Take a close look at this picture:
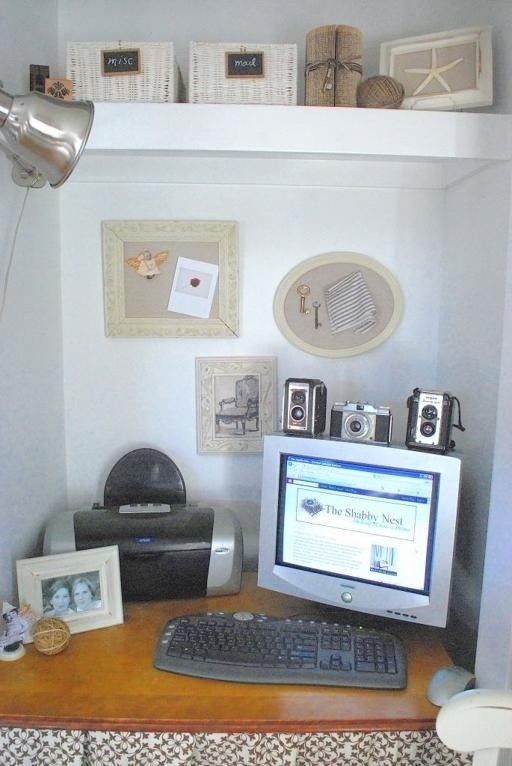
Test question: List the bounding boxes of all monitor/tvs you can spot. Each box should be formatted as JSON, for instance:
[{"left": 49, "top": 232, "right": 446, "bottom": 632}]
[{"left": 257, "top": 432, "right": 466, "bottom": 629}]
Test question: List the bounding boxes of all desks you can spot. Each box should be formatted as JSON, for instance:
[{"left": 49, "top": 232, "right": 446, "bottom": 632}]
[{"left": 1, "top": 570, "right": 466, "bottom": 743}]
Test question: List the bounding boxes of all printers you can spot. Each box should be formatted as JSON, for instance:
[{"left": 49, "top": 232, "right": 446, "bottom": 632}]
[{"left": 43, "top": 448, "right": 243, "bottom": 601}]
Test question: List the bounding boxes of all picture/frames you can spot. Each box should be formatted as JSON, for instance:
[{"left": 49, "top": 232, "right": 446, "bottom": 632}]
[
  {"left": 100, "top": 219, "right": 242, "bottom": 341},
  {"left": 13, "top": 545, "right": 130, "bottom": 646},
  {"left": 193, "top": 355, "right": 280, "bottom": 456},
  {"left": 377, "top": 22, "right": 494, "bottom": 111}
]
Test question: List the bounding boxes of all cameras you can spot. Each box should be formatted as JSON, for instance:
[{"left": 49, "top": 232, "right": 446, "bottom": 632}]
[{"left": 330, "top": 401, "right": 393, "bottom": 445}]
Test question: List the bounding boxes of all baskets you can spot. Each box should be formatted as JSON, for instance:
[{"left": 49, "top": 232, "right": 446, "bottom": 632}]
[
  {"left": 66, "top": 39, "right": 179, "bottom": 108},
  {"left": 187, "top": 40, "right": 298, "bottom": 107}
]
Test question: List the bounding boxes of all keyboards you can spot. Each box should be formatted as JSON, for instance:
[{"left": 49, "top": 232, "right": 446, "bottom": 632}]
[{"left": 152, "top": 612, "right": 407, "bottom": 689}]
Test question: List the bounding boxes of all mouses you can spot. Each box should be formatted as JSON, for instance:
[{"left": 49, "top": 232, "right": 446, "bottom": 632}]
[{"left": 426, "top": 664, "right": 475, "bottom": 708}]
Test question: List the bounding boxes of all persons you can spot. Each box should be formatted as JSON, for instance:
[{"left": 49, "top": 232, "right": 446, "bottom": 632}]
[
  {"left": 0, "top": 600, "right": 33, "bottom": 663},
  {"left": 44, "top": 580, "right": 75, "bottom": 617},
  {"left": 71, "top": 578, "right": 101, "bottom": 613}
]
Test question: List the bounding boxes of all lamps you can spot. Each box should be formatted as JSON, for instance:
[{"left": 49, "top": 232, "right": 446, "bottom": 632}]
[{"left": 1, "top": 84, "right": 101, "bottom": 192}]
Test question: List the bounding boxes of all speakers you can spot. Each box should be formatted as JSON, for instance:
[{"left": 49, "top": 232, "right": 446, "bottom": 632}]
[
  {"left": 283, "top": 378, "right": 326, "bottom": 436},
  {"left": 407, "top": 388, "right": 454, "bottom": 455}
]
